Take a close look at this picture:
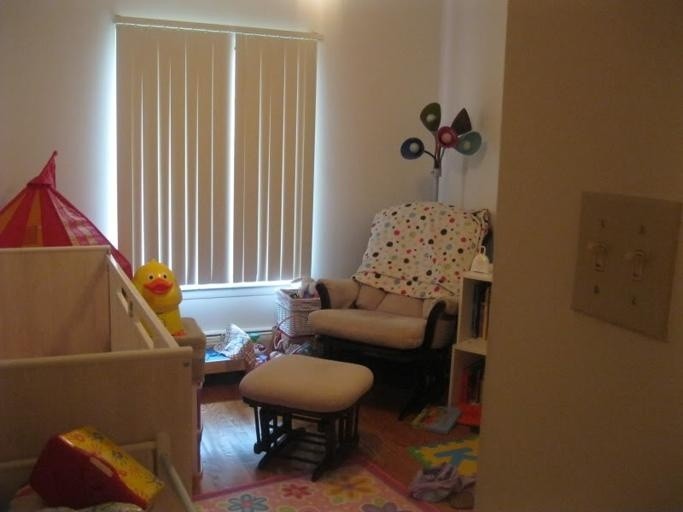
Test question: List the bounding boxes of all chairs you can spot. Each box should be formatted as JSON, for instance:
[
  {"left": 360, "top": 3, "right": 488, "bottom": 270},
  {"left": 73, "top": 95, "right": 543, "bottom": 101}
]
[{"left": 316, "top": 202, "right": 491, "bottom": 421}]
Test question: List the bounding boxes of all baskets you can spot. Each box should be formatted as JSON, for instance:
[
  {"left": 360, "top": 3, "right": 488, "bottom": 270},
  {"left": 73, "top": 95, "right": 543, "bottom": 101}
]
[{"left": 276, "top": 289, "right": 321, "bottom": 337}]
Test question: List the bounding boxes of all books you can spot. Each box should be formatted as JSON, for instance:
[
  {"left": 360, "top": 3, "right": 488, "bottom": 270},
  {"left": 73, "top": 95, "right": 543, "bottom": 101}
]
[
  {"left": 471, "top": 284, "right": 489, "bottom": 343},
  {"left": 465, "top": 355, "right": 486, "bottom": 406},
  {"left": 408, "top": 402, "right": 463, "bottom": 434}
]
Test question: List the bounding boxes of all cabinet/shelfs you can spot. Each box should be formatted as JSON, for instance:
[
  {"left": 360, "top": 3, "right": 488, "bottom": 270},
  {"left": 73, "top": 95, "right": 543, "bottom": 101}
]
[{"left": 446, "top": 270, "right": 491, "bottom": 427}]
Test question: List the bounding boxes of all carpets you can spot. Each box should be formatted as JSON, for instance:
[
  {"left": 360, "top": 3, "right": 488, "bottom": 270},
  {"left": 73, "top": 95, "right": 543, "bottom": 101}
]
[{"left": 191, "top": 453, "right": 442, "bottom": 511}]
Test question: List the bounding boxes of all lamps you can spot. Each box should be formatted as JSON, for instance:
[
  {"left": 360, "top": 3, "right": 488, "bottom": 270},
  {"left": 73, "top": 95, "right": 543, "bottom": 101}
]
[{"left": 399, "top": 101, "right": 482, "bottom": 203}]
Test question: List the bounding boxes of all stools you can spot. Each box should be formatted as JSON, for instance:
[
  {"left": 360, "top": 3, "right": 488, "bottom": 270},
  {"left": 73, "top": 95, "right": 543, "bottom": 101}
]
[{"left": 238, "top": 353, "right": 375, "bottom": 482}]
